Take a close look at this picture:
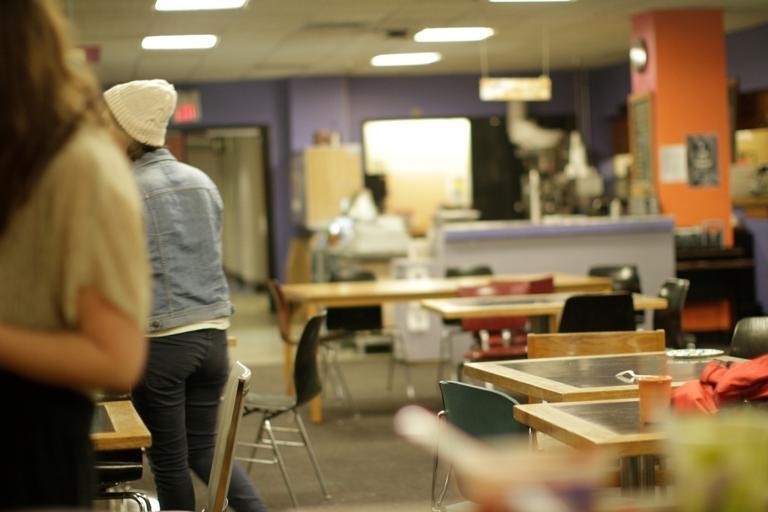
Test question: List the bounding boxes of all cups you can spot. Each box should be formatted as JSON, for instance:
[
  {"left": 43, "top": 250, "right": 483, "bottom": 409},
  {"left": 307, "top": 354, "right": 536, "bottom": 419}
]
[{"left": 637, "top": 375, "right": 673, "bottom": 425}]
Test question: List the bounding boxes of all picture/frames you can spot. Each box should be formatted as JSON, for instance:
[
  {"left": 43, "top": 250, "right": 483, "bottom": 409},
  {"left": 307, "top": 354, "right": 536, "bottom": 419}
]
[{"left": 627, "top": 91, "right": 656, "bottom": 198}]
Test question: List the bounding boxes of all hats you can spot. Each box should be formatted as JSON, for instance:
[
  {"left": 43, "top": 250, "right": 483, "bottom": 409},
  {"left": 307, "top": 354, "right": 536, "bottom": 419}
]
[{"left": 103, "top": 79, "right": 177, "bottom": 147}]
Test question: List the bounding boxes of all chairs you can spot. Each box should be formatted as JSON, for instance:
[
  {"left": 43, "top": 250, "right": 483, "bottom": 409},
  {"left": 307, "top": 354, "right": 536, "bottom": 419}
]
[
  {"left": 322, "top": 269, "right": 410, "bottom": 408},
  {"left": 589, "top": 265, "right": 646, "bottom": 327},
  {"left": 196, "top": 357, "right": 252, "bottom": 511},
  {"left": 223, "top": 311, "right": 330, "bottom": 511},
  {"left": 559, "top": 294, "right": 640, "bottom": 332},
  {"left": 436, "top": 265, "right": 504, "bottom": 396},
  {"left": 651, "top": 276, "right": 691, "bottom": 350},
  {"left": 430, "top": 372, "right": 536, "bottom": 512},
  {"left": 525, "top": 330, "right": 669, "bottom": 358},
  {"left": 270, "top": 274, "right": 362, "bottom": 422}
]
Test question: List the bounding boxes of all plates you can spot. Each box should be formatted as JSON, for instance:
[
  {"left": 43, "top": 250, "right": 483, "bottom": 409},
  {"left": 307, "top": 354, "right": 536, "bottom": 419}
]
[{"left": 666, "top": 348, "right": 725, "bottom": 359}]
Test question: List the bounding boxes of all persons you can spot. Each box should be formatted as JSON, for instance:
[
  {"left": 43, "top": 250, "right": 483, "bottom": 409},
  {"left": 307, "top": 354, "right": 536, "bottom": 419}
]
[
  {"left": 325, "top": 175, "right": 404, "bottom": 252},
  {"left": 0, "top": 0, "right": 152, "bottom": 512},
  {"left": 101, "top": 78, "right": 268, "bottom": 512}
]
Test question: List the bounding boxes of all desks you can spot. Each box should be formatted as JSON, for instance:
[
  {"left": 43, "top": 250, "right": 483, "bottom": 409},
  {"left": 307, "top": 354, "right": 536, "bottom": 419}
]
[
  {"left": 512, "top": 397, "right": 741, "bottom": 512},
  {"left": 461, "top": 347, "right": 746, "bottom": 401}
]
